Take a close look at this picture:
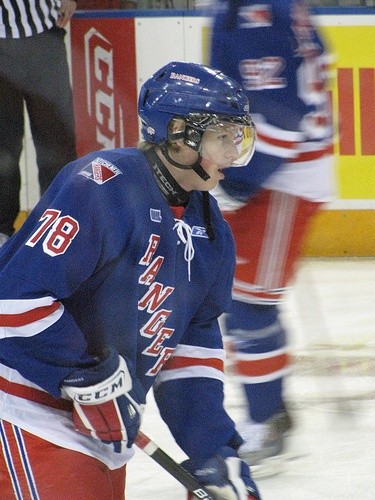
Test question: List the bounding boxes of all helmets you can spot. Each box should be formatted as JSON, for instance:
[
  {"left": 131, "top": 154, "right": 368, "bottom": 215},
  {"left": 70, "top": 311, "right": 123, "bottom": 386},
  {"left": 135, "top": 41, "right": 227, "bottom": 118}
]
[{"left": 138, "top": 60, "right": 257, "bottom": 169}]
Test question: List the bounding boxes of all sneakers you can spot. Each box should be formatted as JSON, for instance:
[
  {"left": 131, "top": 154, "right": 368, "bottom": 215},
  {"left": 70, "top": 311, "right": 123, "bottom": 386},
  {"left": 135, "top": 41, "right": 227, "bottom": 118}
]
[{"left": 234, "top": 411, "right": 312, "bottom": 480}]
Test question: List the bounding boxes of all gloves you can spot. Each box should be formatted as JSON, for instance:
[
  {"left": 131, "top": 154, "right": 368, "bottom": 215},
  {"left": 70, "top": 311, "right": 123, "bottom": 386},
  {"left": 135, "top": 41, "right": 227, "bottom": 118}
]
[
  {"left": 182, "top": 457, "right": 260, "bottom": 500},
  {"left": 63, "top": 344, "right": 141, "bottom": 454}
]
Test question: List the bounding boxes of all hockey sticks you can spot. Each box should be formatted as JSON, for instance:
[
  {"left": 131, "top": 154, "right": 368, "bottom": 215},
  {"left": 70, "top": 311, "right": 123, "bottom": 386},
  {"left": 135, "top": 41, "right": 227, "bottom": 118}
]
[{"left": 135, "top": 430, "right": 216, "bottom": 500}]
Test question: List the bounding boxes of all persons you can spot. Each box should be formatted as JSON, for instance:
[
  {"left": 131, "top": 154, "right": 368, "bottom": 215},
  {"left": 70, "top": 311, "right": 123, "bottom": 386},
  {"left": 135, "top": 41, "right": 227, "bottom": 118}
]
[
  {"left": 0, "top": 63, "right": 261, "bottom": 500},
  {"left": 193, "top": 0, "right": 334, "bottom": 485},
  {"left": 0, "top": 0, "right": 78, "bottom": 245}
]
